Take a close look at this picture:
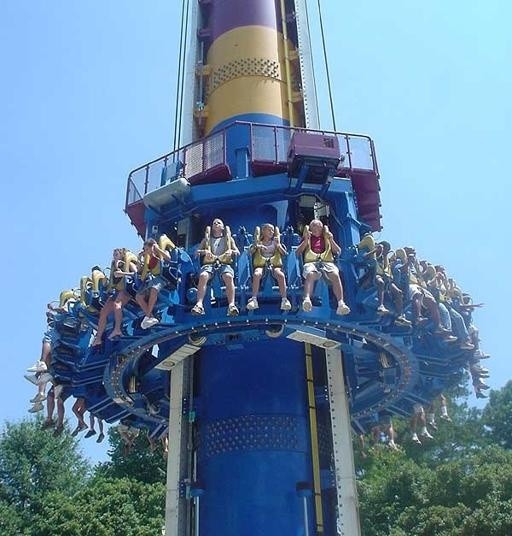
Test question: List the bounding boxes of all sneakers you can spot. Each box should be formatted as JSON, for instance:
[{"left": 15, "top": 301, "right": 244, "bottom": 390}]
[
  {"left": 24, "top": 361, "right": 169, "bottom": 458},
  {"left": 280, "top": 300, "right": 291, "bottom": 310},
  {"left": 246, "top": 298, "right": 259, "bottom": 310},
  {"left": 358, "top": 308, "right": 491, "bottom": 460},
  {"left": 191, "top": 305, "right": 205, "bottom": 315},
  {"left": 302, "top": 297, "right": 313, "bottom": 313},
  {"left": 227, "top": 306, "right": 239, "bottom": 316},
  {"left": 336, "top": 304, "right": 351, "bottom": 316},
  {"left": 141, "top": 316, "right": 159, "bottom": 329}
]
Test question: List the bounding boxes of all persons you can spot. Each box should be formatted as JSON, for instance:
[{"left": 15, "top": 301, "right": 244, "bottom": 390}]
[
  {"left": 354, "top": 241, "right": 491, "bottom": 459},
  {"left": 191, "top": 218, "right": 240, "bottom": 317},
  {"left": 24, "top": 248, "right": 168, "bottom": 460},
  {"left": 295, "top": 220, "right": 351, "bottom": 315},
  {"left": 247, "top": 223, "right": 292, "bottom": 311},
  {"left": 135, "top": 238, "right": 171, "bottom": 330}
]
